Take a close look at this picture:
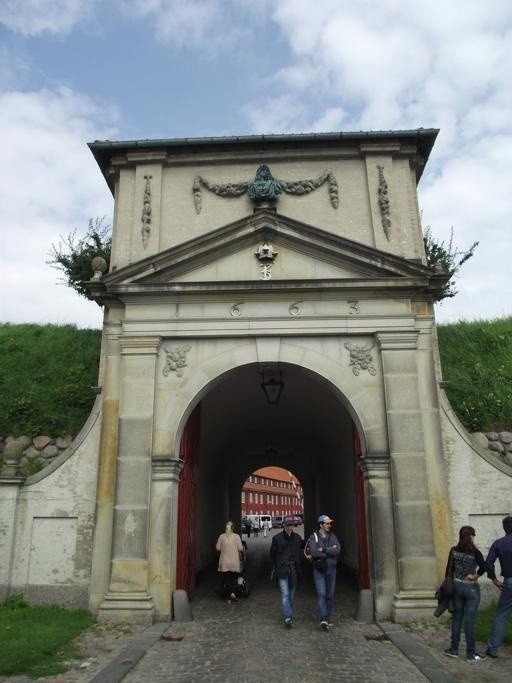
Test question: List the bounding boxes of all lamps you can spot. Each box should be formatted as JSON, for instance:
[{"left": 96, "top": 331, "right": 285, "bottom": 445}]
[
  {"left": 265, "top": 449, "right": 277, "bottom": 463},
  {"left": 261, "top": 369, "right": 286, "bottom": 404}
]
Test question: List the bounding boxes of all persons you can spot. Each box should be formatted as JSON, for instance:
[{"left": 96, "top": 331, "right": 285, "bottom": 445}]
[
  {"left": 486, "top": 516, "right": 512, "bottom": 658},
  {"left": 253, "top": 517, "right": 259, "bottom": 535},
  {"left": 254, "top": 519, "right": 259, "bottom": 538},
  {"left": 445, "top": 526, "right": 487, "bottom": 661},
  {"left": 263, "top": 519, "right": 269, "bottom": 536},
  {"left": 310, "top": 515, "right": 341, "bottom": 630},
  {"left": 246, "top": 518, "right": 252, "bottom": 538},
  {"left": 216, "top": 522, "right": 243, "bottom": 604},
  {"left": 270, "top": 517, "right": 302, "bottom": 625}
]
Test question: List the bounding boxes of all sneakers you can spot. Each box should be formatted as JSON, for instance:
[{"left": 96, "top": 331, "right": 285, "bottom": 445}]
[
  {"left": 467, "top": 652, "right": 487, "bottom": 663},
  {"left": 284, "top": 617, "right": 293, "bottom": 624},
  {"left": 443, "top": 648, "right": 459, "bottom": 658},
  {"left": 320, "top": 620, "right": 329, "bottom": 632}
]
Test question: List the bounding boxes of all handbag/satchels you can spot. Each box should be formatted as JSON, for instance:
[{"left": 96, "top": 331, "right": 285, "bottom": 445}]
[{"left": 303, "top": 532, "right": 323, "bottom": 563}]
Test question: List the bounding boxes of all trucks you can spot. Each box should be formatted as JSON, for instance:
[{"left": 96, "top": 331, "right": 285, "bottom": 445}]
[{"left": 243, "top": 514, "right": 273, "bottom": 530}]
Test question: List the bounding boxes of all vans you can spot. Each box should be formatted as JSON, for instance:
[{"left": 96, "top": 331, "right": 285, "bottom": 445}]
[{"left": 272, "top": 516, "right": 285, "bottom": 528}]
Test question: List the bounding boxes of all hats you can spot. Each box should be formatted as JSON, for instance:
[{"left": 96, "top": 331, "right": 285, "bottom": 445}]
[
  {"left": 317, "top": 514, "right": 334, "bottom": 524},
  {"left": 284, "top": 515, "right": 294, "bottom": 526}
]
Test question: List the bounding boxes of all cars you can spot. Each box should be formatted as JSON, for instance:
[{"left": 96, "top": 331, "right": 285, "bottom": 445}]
[
  {"left": 285, "top": 516, "right": 302, "bottom": 526},
  {"left": 241, "top": 520, "right": 254, "bottom": 532}
]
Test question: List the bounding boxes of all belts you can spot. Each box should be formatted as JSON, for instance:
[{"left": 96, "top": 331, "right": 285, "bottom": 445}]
[{"left": 454, "top": 578, "right": 477, "bottom": 583}]
